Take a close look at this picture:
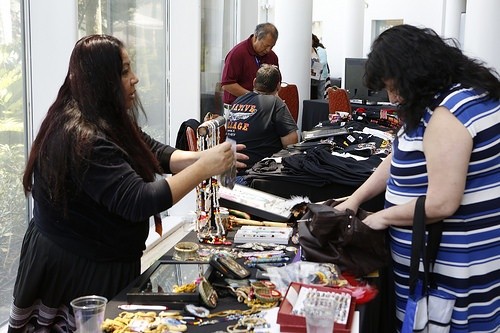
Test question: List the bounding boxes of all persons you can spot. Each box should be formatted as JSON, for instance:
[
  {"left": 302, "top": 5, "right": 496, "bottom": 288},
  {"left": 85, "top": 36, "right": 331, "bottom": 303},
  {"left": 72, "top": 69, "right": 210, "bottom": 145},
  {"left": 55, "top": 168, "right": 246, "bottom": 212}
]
[
  {"left": 8, "top": 34, "right": 249, "bottom": 333},
  {"left": 311, "top": 34, "right": 328, "bottom": 100},
  {"left": 219, "top": 23, "right": 279, "bottom": 104},
  {"left": 334, "top": 24, "right": 500, "bottom": 333},
  {"left": 228, "top": 64, "right": 299, "bottom": 176}
]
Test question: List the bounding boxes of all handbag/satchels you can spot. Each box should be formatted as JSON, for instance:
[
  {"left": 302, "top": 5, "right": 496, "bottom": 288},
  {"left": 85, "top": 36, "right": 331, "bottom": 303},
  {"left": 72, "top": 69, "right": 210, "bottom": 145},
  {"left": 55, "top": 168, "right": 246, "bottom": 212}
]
[
  {"left": 399, "top": 195, "right": 456, "bottom": 332},
  {"left": 295, "top": 198, "right": 394, "bottom": 278}
]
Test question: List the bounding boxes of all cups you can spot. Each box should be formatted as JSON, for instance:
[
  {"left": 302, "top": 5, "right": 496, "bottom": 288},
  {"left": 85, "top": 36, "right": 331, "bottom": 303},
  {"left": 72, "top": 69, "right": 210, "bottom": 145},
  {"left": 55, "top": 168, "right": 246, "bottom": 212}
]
[
  {"left": 302, "top": 298, "right": 336, "bottom": 333},
  {"left": 70, "top": 296, "right": 108, "bottom": 333}
]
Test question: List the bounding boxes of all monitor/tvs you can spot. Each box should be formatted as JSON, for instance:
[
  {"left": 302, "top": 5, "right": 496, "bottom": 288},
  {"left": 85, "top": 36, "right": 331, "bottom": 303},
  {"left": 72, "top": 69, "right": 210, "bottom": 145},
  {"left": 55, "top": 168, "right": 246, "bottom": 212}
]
[{"left": 344, "top": 58, "right": 390, "bottom": 102}]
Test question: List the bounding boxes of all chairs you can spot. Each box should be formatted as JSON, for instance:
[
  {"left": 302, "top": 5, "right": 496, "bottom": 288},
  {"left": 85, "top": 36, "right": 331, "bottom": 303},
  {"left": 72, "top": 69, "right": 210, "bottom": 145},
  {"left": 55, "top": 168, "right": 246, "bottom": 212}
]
[
  {"left": 328, "top": 85, "right": 352, "bottom": 116},
  {"left": 278, "top": 82, "right": 299, "bottom": 124}
]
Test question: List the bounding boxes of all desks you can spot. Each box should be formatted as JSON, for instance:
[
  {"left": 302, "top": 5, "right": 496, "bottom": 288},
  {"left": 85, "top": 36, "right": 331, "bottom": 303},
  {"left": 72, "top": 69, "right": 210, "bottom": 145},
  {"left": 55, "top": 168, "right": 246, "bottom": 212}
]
[
  {"left": 300, "top": 99, "right": 398, "bottom": 143},
  {"left": 74, "top": 225, "right": 365, "bottom": 333}
]
